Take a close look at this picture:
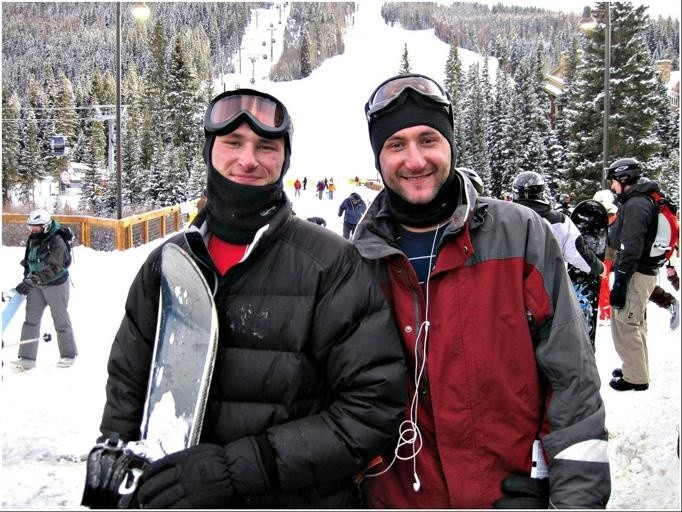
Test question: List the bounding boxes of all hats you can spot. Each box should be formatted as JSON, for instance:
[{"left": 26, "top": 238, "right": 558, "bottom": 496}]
[
  {"left": 204, "top": 134, "right": 289, "bottom": 244},
  {"left": 369, "top": 98, "right": 460, "bottom": 227}
]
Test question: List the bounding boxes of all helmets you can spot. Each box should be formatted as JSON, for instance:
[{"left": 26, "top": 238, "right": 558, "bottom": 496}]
[
  {"left": 559, "top": 193, "right": 570, "bottom": 205},
  {"left": 593, "top": 189, "right": 615, "bottom": 210},
  {"left": 27, "top": 209, "right": 51, "bottom": 234},
  {"left": 512, "top": 171, "right": 544, "bottom": 200},
  {"left": 607, "top": 158, "right": 641, "bottom": 185}
]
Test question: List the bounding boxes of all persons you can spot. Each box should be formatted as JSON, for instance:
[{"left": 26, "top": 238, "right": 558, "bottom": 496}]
[
  {"left": 284, "top": 173, "right": 367, "bottom": 238},
  {"left": 90, "top": 89, "right": 408, "bottom": 510},
  {"left": 512, "top": 156, "right": 680, "bottom": 391},
  {"left": 12, "top": 208, "right": 78, "bottom": 368},
  {"left": 347, "top": 73, "right": 611, "bottom": 510}
]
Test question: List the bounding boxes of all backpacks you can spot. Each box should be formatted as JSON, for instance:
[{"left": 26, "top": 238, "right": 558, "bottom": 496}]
[{"left": 615, "top": 194, "right": 679, "bottom": 269}]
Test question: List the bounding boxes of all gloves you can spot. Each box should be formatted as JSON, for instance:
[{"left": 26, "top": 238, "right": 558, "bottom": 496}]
[
  {"left": 15, "top": 275, "right": 42, "bottom": 295},
  {"left": 609, "top": 266, "right": 635, "bottom": 309},
  {"left": 494, "top": 477, "right": 549, "bottom": 510},
  {"left": 136, "top": 436, "right": 270, "bottom": 509}
]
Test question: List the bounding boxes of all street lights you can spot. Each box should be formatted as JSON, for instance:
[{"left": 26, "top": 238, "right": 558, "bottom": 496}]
[{"left": 112, "top": 1, "right": 150, "bottom": 224}]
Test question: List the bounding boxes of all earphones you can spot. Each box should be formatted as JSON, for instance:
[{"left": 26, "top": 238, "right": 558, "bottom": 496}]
[{"left": 413, "top": 471, "right": 420, "bottom": 492}]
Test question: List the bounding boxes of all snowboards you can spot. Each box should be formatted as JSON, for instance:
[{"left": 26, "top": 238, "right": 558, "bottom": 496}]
[
  {"left": 2, "top": 288, "right": 25, "bottom": 335},
  {"left": 79, "top": 243, "right": 221, "bottom": 506},
  {"left": 567, "top": 200, "right": 608, "bottom": 357}
]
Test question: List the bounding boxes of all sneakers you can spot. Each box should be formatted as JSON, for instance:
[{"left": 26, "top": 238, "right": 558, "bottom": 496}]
[
  {"left": 610, "top": 369, "right": 649, "bottom": 391},
  {"left": 57, "top": 357, "right": 75, "bottom": 367},
  {"left": 666, "top": 299, "right": 680, "bottom": 329},
  {"left": 10, "top": 359, "right": 35, "bottom": 370}
]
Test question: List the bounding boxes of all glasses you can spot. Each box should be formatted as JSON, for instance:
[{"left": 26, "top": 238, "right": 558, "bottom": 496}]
[
  {"left": 604, "top": 164, "right": 640, "bottom": 180},
  {"left": 365, "top": 74, "right": 454, "bottom": 132},
  {"left": 204, "top": 89, "right": 292, "bottom": 153}
]
